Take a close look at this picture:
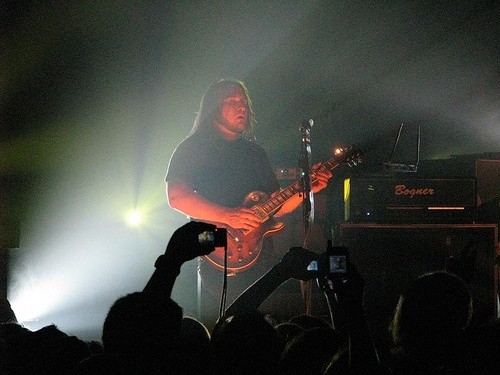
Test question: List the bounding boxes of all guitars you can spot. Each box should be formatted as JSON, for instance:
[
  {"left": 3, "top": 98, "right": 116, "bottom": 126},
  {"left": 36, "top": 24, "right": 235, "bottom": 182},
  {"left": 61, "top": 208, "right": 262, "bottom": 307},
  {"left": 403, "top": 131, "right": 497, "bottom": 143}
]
[{"left": 201, "top": 142, "right": 367, "bottom": 274}]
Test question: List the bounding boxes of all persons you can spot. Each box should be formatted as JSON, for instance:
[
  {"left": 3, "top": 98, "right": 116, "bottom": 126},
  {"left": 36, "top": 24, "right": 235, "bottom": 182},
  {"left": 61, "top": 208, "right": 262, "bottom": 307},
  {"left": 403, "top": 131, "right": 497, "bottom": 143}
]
[
  {"left": 1, "top": 296, "right": 103, "bottom": 375},
  {"left": 181, "top": 244, "right": 500, "bottom": 375},
  {"left": 164, "top": 77, "right": 333, "bottom": 326},
  {"left": 102, "top": 220, "right": 218, "bottom": 375}
]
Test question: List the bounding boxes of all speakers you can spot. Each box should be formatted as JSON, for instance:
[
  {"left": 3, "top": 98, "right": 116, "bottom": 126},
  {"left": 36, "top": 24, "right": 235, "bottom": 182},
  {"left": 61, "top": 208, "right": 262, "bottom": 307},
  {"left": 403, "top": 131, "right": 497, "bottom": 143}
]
[{"left": 335, "top": 220, "right": 499, "bottom": 339}]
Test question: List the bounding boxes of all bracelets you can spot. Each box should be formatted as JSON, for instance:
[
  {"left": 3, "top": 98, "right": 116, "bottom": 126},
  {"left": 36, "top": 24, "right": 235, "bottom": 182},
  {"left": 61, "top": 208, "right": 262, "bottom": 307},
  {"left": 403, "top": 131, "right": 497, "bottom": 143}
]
[{"left": 153, "top": 255, "right": 182, "bottom": 277}]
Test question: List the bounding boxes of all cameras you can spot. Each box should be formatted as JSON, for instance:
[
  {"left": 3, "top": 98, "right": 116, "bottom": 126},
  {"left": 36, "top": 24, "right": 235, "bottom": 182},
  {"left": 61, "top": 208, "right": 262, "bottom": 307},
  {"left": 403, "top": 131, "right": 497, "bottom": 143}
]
[
  {"left": 197, "top": 228, "right": 227, "bottom": 247},
  {"left": 305, "top": 253, "right": 349, "bottom": 273}
]
